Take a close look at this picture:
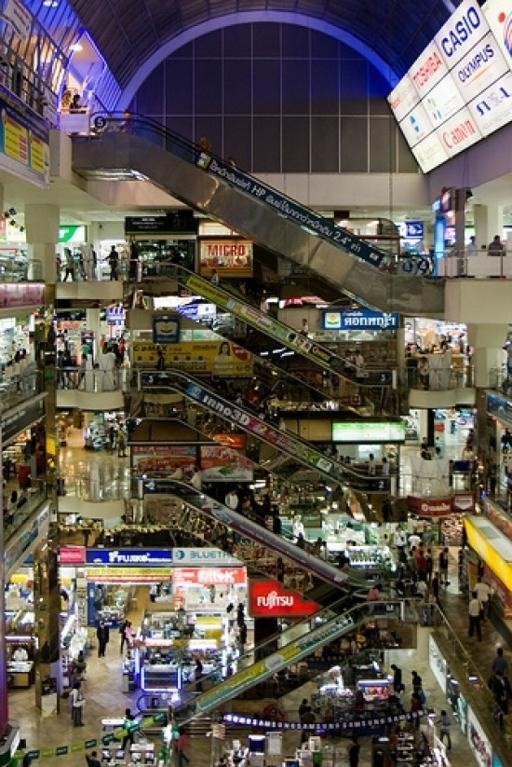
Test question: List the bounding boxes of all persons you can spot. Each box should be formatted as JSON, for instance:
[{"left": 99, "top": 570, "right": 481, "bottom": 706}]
[
  {"left": 119, "top": 107, "right": 132, "bottom": 133},
  {"left": 11, "top": 233, "right": 511, "bottom": 767},
  {"left": 227, "top": 156, "right": 235, "bottom": 167},
  {"left": 195, "top": 134, "right": 212, "bottom": 151},
  {"left": 69, "top": 94, "right": 80, "bottom": 136}
]
